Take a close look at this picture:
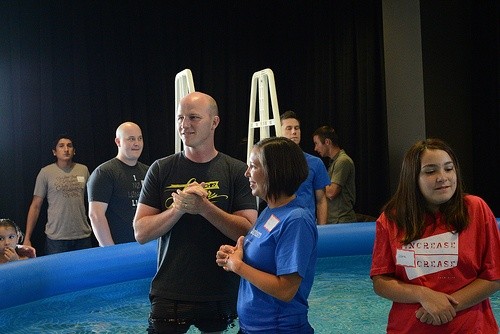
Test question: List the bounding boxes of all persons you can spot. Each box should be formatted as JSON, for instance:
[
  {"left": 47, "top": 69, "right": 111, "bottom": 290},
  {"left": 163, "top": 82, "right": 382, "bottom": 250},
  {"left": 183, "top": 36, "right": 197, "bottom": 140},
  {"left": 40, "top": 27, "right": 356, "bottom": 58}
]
[
  {"left": 370, "top": 139, "right": 500, "bottom": 334},
  {"left": 22, "top": 135, "right": 92, "bottom": 256},
  {"left": 86, "top": 122, "right": 151, "bottom": 247},
  {"left": 313, "top": 125, "right": 358, "bottom": 224},
  {"left": 215, "top": 137, "right": 319, "bottom": 334},
  {"left": 132, "top": 91, "right": 258, "bottom": 334},
  {"left": 0, "top": 218, "right": 36, "bottom": 264},
  {"left": 280, "top": 111, "right": 331, "bottom": 225}
]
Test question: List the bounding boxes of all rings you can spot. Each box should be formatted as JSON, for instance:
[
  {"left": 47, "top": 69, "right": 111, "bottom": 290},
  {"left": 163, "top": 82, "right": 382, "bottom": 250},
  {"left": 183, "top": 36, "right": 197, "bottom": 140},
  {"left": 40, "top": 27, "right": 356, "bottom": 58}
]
[{"left": 185, "top": 204, "right": 188, "bottom": 208}]
